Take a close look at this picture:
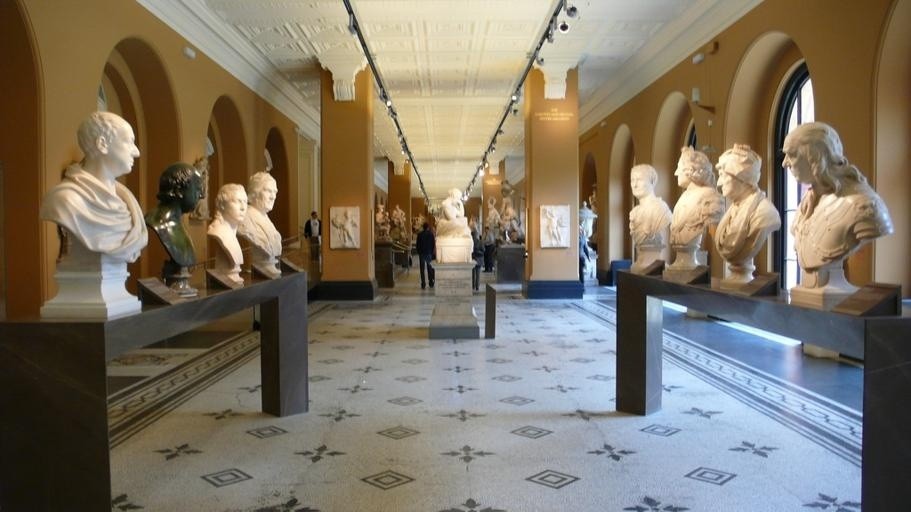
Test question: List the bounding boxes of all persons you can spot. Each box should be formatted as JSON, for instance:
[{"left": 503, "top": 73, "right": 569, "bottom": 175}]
[
  {"left": 39, "top": 111, "right": 282, "bottom": 267},
  {"left": 471, "top": 226, "right": 495, "bottom": 292},
  {"left": 304, "top": 212, "right": 322, "bottom": 264},
  {"left": 579, "top": 234, "right": 591, "bottom": 292},
  {"left": 376, "top": 204, "right": 406, "bottom": 240},
  {"left": 328, "top": 209, "right": 363, "bottom": 248},
  {"left": 544, "top": 207, "right": 568, "bottom": 246},
  {"left": 484, "top": 180, "right": 525, "bottom": 244},
  {"left": 416, "top": 222, "right": 437, "bottom": 289},
  {"left": 579, "top": 200, "right": 598, "bottom": 239},
  {"left": 440, "top": 188, "right": 465, "bottom": 221}
]
